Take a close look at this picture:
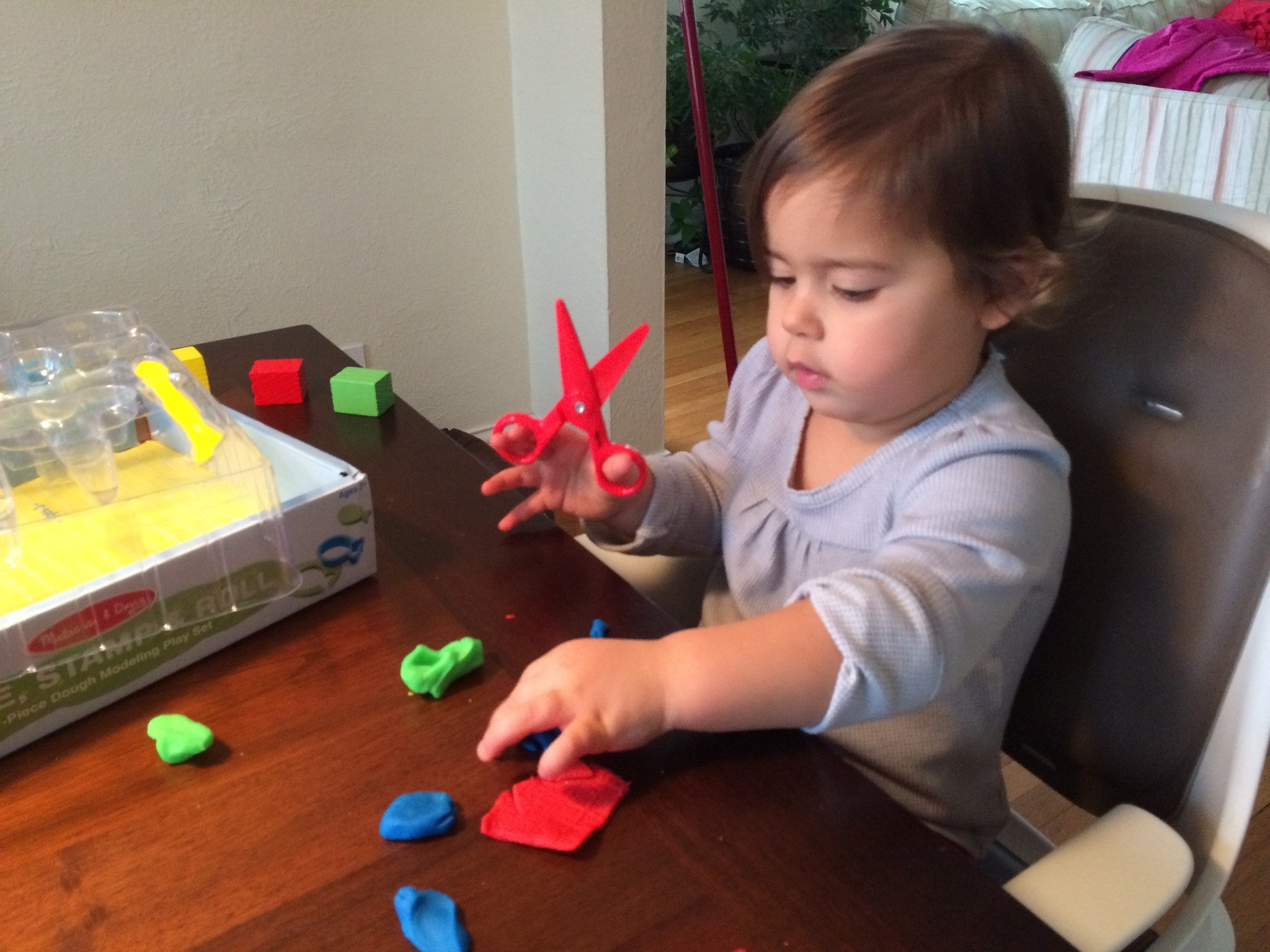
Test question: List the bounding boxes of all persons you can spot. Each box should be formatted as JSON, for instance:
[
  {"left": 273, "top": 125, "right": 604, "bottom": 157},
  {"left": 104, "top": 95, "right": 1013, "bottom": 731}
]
[{"left": 475, "top": 21, "right": 1075, "bottom": 883}]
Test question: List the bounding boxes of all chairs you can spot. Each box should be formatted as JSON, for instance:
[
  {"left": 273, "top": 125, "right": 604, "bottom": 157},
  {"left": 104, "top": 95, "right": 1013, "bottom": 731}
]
[{"left": 574, "top": 182, "right": 1270, "bottom": 952}]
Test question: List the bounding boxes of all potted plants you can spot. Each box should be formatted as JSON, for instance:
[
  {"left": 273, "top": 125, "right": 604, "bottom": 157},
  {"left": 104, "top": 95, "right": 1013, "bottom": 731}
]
[{"left": 666, "top": 1, "right": 912, "bottom": 273}]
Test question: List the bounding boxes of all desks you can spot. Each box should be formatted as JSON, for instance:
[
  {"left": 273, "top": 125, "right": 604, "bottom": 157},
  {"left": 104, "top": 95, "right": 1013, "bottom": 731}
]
[{"left": 0, "top": 325, "right": 1084, "bottom": 952}]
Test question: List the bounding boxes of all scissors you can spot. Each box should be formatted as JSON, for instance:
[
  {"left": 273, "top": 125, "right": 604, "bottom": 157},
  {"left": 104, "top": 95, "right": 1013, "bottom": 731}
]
[{"left": 491, "top": 298, "right": 651, "bottom": 499}]
